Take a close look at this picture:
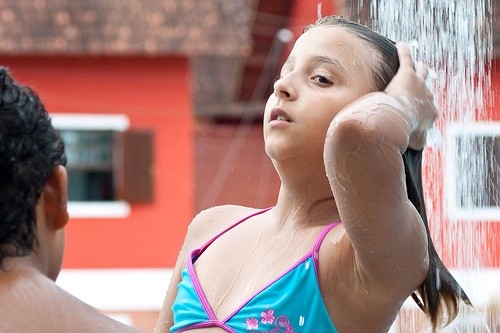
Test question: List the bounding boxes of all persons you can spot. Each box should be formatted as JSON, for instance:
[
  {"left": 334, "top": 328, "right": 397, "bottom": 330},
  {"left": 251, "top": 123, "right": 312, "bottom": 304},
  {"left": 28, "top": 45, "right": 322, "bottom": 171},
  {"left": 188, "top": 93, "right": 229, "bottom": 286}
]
[
  {"left": 0, "top": 66, "right": 140, "bottom": 332},
  {"left": 154, "top": 16, "right": 473, "bottom": 333}
]
